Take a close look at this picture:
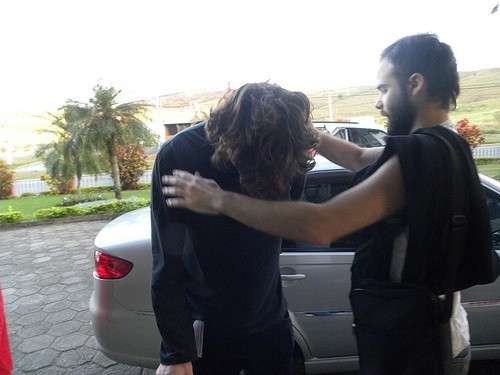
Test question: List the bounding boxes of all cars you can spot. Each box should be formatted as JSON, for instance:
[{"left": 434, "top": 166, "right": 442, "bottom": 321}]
[
  {"left": 88, "top": 161, "right": 500, "bottom": 374},
  {"left": 306, "top": 121, "right": 391, "bottom": 171}
]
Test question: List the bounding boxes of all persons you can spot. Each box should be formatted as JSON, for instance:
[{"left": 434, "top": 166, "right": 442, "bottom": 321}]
[
  {"left": 161, "top": 32, "right": 500, "bottom": 375},
  {"left": 148, "top": 81, "right": 318, "bottom": 375}
]
